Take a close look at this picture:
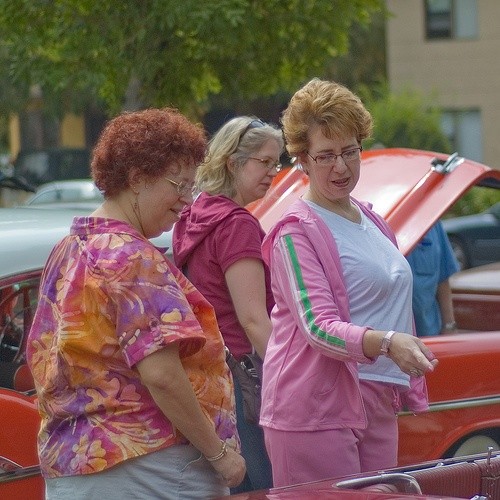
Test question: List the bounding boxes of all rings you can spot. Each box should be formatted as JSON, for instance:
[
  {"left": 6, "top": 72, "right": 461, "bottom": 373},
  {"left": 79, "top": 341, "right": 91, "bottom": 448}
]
[{"left": 413, "top": 369, "right": 423, "bottom": 376}]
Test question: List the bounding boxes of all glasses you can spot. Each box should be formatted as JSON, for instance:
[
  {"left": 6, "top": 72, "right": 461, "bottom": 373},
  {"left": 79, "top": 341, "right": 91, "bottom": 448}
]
[
  {"left": 248, "top": 156, "right": 282, "bottom": 173},
  {"left": 162, "top": 176, "right": 197, "bottom": 197},
  {"left": 305, "top": 146, "right": 362, "bottom": 166},
  {"left": 238, "top": 120, "right": 279, "bottom": 145}
]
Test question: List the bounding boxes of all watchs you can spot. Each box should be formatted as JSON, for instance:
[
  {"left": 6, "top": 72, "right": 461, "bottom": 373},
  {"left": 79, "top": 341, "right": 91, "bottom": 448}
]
[
  {"left": 205, "top": 439, "right": 228, "bottom": 462},
  {"left": 442, "top": 321, "right": 456, "bottom": 330},
  {"left": 380, "top": 331, "right": 395, "bottom": 357}
]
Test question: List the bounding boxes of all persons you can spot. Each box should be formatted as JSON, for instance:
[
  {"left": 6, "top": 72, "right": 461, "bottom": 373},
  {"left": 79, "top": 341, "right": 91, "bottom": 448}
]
[
  {"left": 259, "top": 78, "right": 437, "bottom": 489},
  {"left": 173, "top": 116, "right": 285, "bottom": 495},
  {"left": 25, "top": 105, "right": 247, "bottom": 500},
  {"left": 405, "top": 219, "right": 460, "bottom": 337}
]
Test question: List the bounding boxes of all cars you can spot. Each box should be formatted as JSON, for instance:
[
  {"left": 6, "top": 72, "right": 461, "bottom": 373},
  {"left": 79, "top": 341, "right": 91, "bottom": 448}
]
[
  {"left": 439, "top": 197, "right": 500, "bottom": 271},
  {"left": 15, "top": 175, "right": 106, "bottom": 211},
  {"left": 445, "top": 260, "right": 500, "bottom": 331},
  {"left": 0, "top": 146, "right": 500, "bottom": 500}
]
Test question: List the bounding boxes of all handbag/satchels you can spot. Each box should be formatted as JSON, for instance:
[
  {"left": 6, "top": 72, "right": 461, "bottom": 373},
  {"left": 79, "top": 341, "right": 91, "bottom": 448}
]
[{"left": 234, "top": 354, "right": 264, "bottom": 425}]
